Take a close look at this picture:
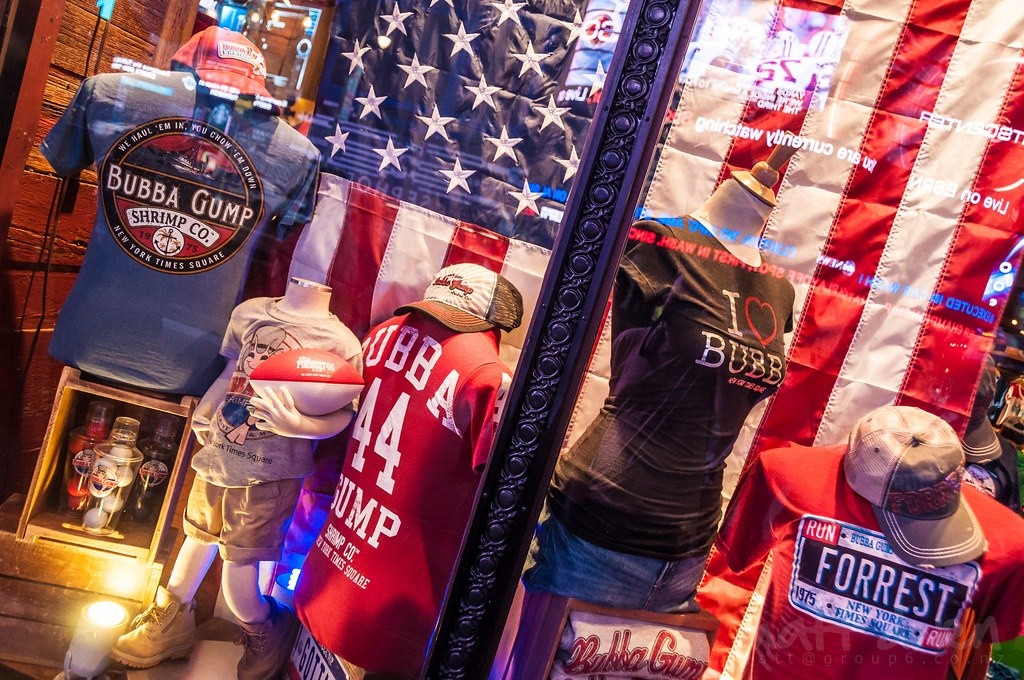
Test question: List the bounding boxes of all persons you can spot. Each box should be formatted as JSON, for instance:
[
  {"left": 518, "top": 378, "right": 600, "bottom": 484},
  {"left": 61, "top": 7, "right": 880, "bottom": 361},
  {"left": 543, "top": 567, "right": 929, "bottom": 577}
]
[
  {"left": 292, "top": 263, "right": 513, "bottom": 675},
  {"left": 111, "top": 278, "right": 361, "bottom": 680},
  {"left": 38, "top": 26, "right": 319, "bottom": 396},
  {"left": 715, "top": 408, "right": 1023, "bottom": 680},
  {"left": 522, "top": 177, "right": 795, "bottom": 614}
]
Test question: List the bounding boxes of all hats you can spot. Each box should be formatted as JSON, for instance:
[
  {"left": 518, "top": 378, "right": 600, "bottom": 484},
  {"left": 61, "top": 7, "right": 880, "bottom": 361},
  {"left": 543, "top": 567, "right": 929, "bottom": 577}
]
[
  {"left": 960, "top": 354, "right": 1002, "bottom": 464},
  {"left": 171, "top": 26, "right": 273, "bottom": 99},
  {"left": 843, "top": 407, "right": 987, "bottom": 569},
  {"left": 393, "top": 262, "right": 524, "bottom": 334}
]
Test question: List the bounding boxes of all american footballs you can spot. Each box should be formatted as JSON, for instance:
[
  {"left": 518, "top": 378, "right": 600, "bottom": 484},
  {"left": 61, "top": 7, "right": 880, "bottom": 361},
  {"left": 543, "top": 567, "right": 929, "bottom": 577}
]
[{"left": 249, "top": 347, "right": 365, "bottom": 415}]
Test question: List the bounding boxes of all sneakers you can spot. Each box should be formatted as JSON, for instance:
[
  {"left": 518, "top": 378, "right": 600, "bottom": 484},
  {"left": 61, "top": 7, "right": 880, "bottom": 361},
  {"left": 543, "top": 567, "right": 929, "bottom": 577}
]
[
  {"left": 234, "top": 596, "right": 296, "bottom": 680},
  {"left": 110, "top": 585, "right": 197, "bottom": 669}
]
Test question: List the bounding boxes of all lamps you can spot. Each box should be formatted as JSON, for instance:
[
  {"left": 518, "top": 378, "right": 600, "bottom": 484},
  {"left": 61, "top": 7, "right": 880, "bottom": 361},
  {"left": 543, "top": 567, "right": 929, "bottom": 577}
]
[{"left": 63, "top": 600, "right": 130, "bottom": 680}]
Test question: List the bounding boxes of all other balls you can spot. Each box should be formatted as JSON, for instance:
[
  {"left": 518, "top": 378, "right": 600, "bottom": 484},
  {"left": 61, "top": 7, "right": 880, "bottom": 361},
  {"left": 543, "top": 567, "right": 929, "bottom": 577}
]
[{"left": 66, "top": 422, "right": 134, "bottom": 529}]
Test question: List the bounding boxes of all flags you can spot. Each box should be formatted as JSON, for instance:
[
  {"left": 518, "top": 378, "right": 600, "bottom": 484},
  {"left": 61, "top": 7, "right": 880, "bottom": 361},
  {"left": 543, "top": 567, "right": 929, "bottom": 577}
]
[{"left": 263, "top": 2, "right": 1024, "bottom": 678}]
